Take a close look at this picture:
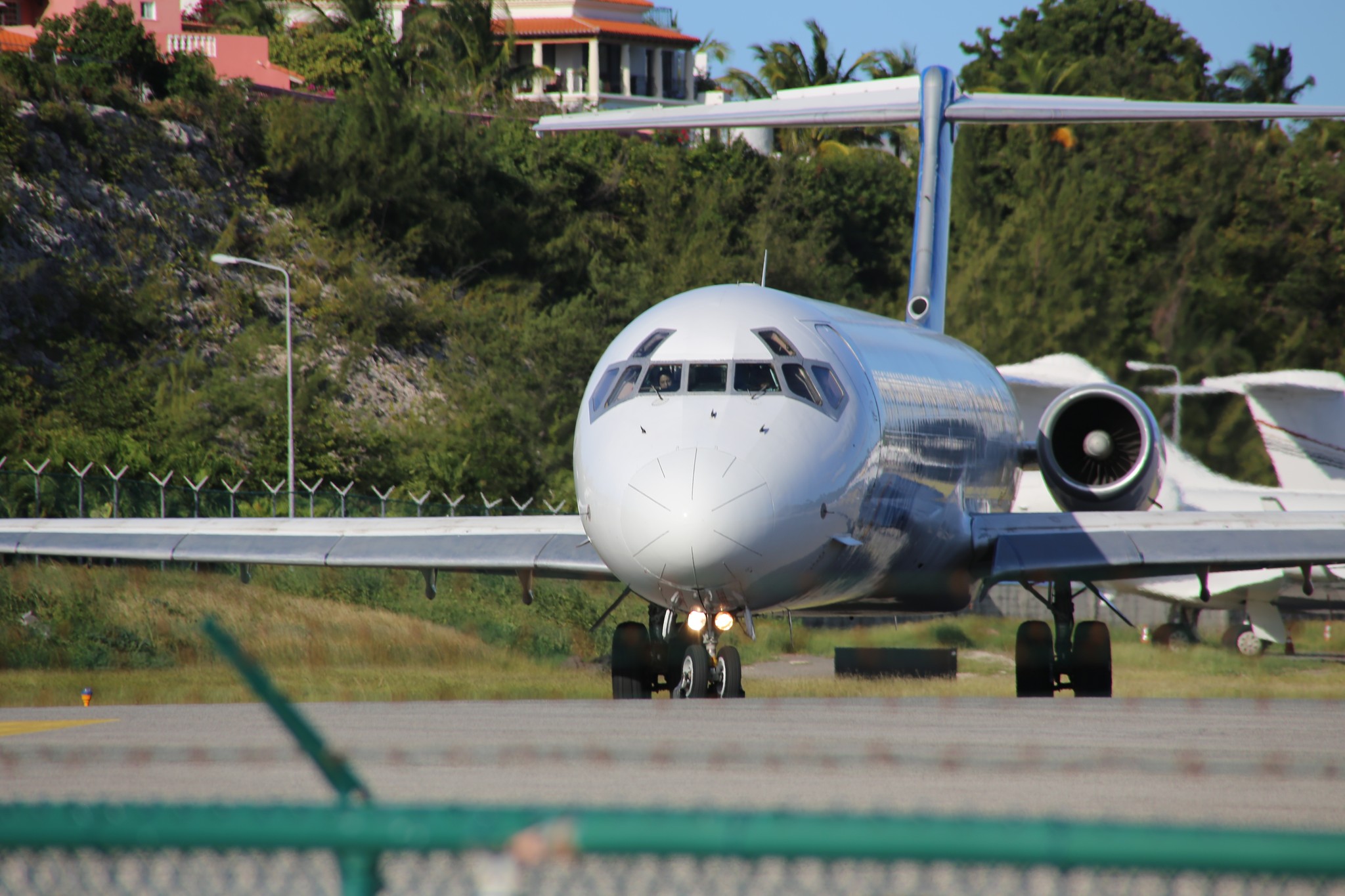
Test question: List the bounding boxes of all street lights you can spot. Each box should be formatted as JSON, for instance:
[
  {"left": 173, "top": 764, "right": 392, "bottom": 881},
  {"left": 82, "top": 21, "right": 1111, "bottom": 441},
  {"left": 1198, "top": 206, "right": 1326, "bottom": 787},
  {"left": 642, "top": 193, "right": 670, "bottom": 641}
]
[
  {"left": 210, "top": 252, "right": 294, "bottom": 519},
  {"left": 1125, "top": 360, "right": 1181, "bottom": 445}
]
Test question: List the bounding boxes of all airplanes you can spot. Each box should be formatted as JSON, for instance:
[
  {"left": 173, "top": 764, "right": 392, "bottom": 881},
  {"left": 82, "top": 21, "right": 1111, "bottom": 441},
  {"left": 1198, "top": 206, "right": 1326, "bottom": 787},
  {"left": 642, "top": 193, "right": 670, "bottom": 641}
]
[{"left": 0, "top": 66, "right": 1345, "bottom": 701}]
